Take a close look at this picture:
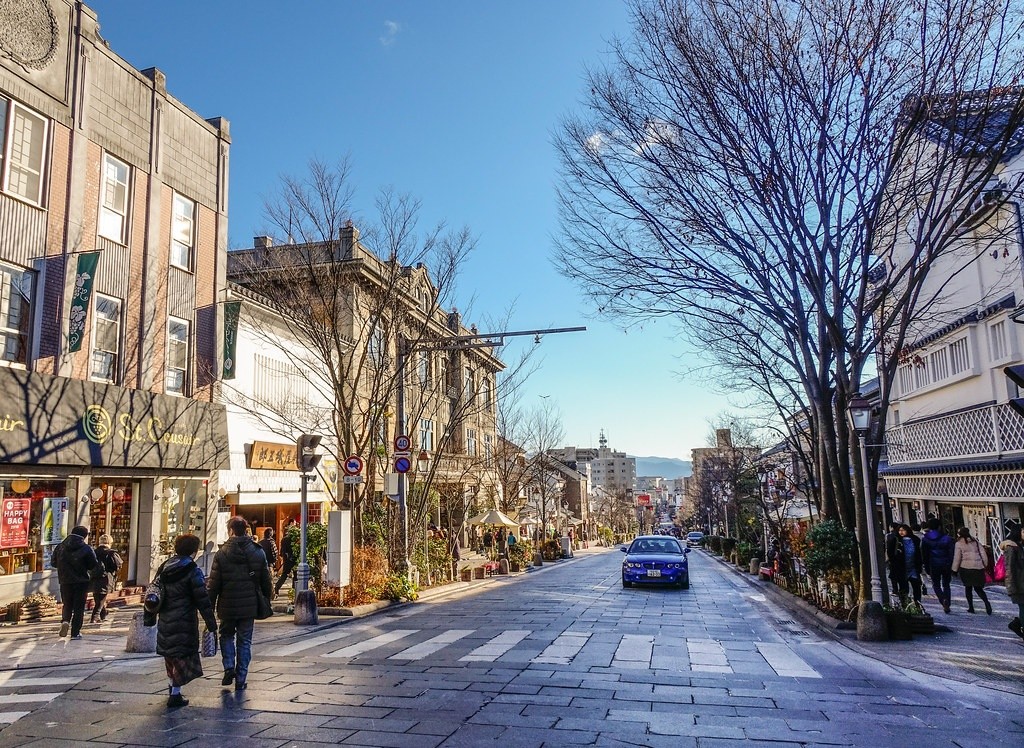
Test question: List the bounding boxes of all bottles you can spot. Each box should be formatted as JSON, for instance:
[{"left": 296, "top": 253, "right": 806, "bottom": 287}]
[
  {"left": 18, "top": 558, "right": 29, "bottom": 572},
  {"left": 88, "top": 514, "right": 130, "bottom": 556},
  {"left": 44, "top": 500, "right": 53, "bottom": 541},
  {"left": 58, "top": 502, "right": 67, "bottom": 539}
]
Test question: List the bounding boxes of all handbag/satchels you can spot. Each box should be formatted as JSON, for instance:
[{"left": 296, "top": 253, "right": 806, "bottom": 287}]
[
  {"left": 984, "top": 570, "right": 992, "bottom": 583},
  {"left": 201, "top": 625, "right": 218, "bottom": 656},
  {"left": 255, "top": 589, "right": 273, "bottom": 619},
  {"left": 993, "top": 553, "right": 1005, "bottom": 580},
  {"left": 144, "top": 570, "right": 163, "bottom": 612}
]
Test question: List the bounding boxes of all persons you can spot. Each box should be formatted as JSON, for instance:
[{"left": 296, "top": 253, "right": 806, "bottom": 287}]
[
  {"left": 495, "top": 526, "right": 507, "bottom": 543},
  {"left": 477, "top": 525, "right": 482, "bottom": 543},
  {"left": 247, "top": 518, "right": 258, "bottom": 534},
  {"left": 427, "top": 524, "right": 460, "bottom": 560},
  {"left": 519, "top": 527, "right": 528, "bottom": 537},
  {"left": 546, "top": 529, "right": 549, "bottom": 539},
  {"left": 554, "top": 528, "right": 588, "bottom": 549},
  {"left": 245, "top": 525, "right": 253, "bottom": 539},
  {"left": 252, "top": 535, "right": 258, "bottom": 542},
  {"left": 508, "top": 530, "right": 517, "bottom": 553},
  {"left": 702, "top": 525, "right": 798, "bottom": 593},
  {"left": 275, "top": 527, "right": 297, "bottom": 599},
  {"left": 207, "top": 515, "right": 273, "bottom": 691},
  {"left": 637, "top": 541, "right": 654, "bottom": 551},
  {"left": 533, "top": 527, "right": 541, "bottom": 545},
  {"left": 259, "top": 527, "right": 279, "bottom": 600},
  {"left": 653, "top": 526, "right": 684, "bottom": 540},
  {"left": 483, "top": 528, "right": 496, "bottom": 559},
  {"left": 51, "top": 525, "right": 98, "bottom": 640},
  {"left": 284, "top": 518, "right": 296, "bottom": 537},
  {"left": 886, "top": 518, "right": 1024, "bottom": 639},
  {"left": 90, "top": 533, "right": 124, "bottom": 623},
  {"left": 144, "top": 534, "right": 217, "bottom": 708},
  {"left": 538, "top": 527, "right": 543, "bottom": 541}
]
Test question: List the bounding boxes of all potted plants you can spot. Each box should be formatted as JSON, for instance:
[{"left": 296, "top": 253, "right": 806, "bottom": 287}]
[
  {"left": 882, "top": 603, "right": 912, "bottom": 639},
  {"left": 905, "top": 596, "right": 934, "bottom": 634}
]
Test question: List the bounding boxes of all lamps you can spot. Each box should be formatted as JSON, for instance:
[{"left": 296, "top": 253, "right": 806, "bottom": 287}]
[
  {"left": 155, "top": 488, "right": 173, "bottom": 499},
  {"left": 211, "top": 488, "right": 226, "bottom": 498},
  {"left": 82, "top": 488, "right": 104, "bottom": 506}
]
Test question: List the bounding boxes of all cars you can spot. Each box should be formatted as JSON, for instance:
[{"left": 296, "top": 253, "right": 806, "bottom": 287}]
[
  {"left": 619, "top": 535, "right": 691, "bottom": 590},
  {"left": 685, "top": 531, "right": 704, "bottom": 549}
]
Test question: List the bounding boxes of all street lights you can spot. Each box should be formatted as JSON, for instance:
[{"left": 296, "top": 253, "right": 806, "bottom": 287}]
[{"left": 843, "top": 391, "right": 889, "bottom": 610}]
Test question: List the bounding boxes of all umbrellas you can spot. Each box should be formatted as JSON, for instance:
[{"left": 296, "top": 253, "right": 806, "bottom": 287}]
[
  {"left": 518, "top": 516, "right": 542, "bottom": 525},
  {"left": 759, "top": 498, "right": 821, "bottom": 525},
  {"left": 465, "top": 510, "right": 522, "bottom": 561}
]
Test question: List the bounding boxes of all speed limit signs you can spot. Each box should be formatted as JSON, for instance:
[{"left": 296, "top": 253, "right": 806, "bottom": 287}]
[{"left": 395, "top": 435, "right": 411, "bottom": 451}]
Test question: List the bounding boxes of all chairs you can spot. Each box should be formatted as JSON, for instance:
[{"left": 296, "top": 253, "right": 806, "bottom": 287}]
[{"left": 482, "top": 561, "right": 500, "bottom": 577}]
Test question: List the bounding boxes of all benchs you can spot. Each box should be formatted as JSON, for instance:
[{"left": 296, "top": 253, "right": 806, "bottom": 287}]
[{"left": 760, "top": 567, "right": 775, "bottom": 581}]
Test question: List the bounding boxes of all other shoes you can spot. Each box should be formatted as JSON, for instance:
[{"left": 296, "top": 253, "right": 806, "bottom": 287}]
[
  {"left": 166, "top": 693, "right": 189, "bottom": 706},
  {"left": 273, "top": 594, "right": 279, "bottom": 601},
  {"left": 101, "top": 611, "right": 108, "bottom": 620},
  {"left": 90, "top": 619, "right": 101, "bottom": 623},
  {"left": 891, "top": 593, "right": 899, "bottom": 597},
  {"left": 967, "top": 608, "right": 975, "bottom": 613},
  {"left": 235, "top": 683, "right": 247, "bottom": 689},
  {"left": 943, "top": 600, "right": 951, "bottom": 613},
  {"left": 60, "top": 622, "right": 69, "bottom": 636},
  {"left": 70, "top": 634, "right": 81, "bottom": 640},
  {"left": 168, "top": 684, "right": 172, "bottom": 694},
  {"left": 222, "top": 668, "right": 236, "bottom": 686},
  {"left": 1008, "top": 617, "right": 1024, "bottom": 638},
  {"left": 985, "top": 601, "right": 992, "bottom": 615}
]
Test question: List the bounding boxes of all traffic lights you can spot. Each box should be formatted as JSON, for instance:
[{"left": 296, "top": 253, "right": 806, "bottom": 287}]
[{"left": 297, "top": 434, "right": 323, "bottom": 473}]
